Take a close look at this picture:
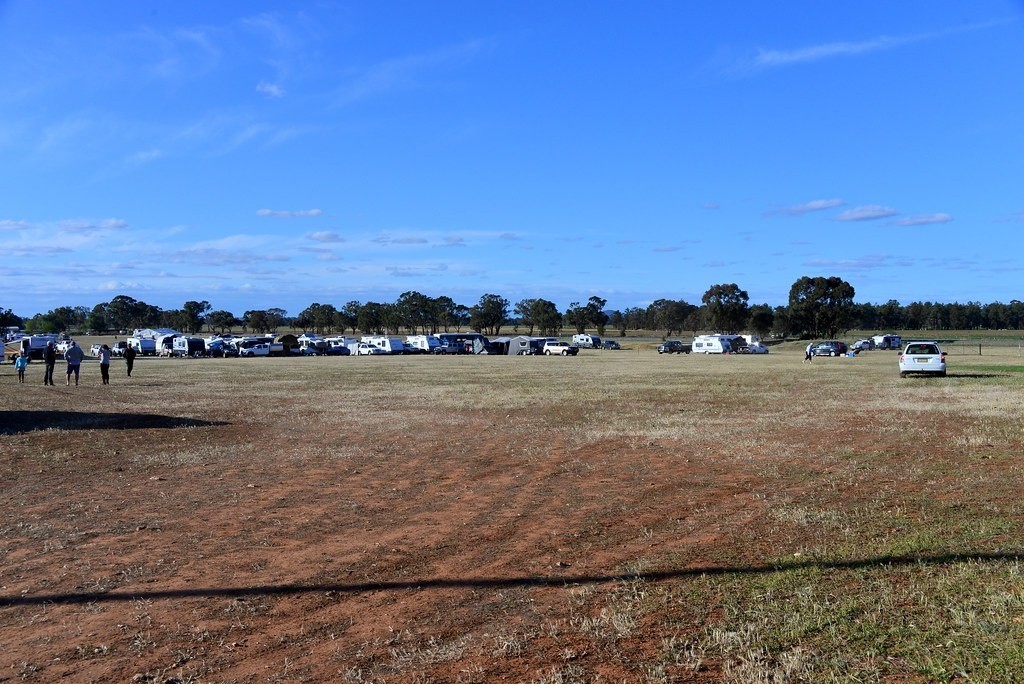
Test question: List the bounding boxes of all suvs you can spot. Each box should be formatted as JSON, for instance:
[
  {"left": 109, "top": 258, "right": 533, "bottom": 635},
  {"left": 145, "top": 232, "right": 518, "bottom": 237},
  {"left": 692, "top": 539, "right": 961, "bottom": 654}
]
[
  {"left": 433, "top": 341, "right": 464, "bottom": 355},
  {"left": 812, "top": 341, "right": 848, "bottom": 357}
]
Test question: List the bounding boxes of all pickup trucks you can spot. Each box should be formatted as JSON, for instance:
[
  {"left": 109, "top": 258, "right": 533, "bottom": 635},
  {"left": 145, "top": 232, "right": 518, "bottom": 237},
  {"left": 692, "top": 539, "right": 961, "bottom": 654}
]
[
  {"left": 656, "top": 341, "right": 692, "bottom": 354},
  {"left": 600, "top": 339, "right": 621, "bottom": 350}
]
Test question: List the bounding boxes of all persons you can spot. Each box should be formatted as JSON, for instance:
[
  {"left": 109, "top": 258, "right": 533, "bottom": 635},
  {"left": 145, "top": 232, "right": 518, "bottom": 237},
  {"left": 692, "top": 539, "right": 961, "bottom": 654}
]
[
  {"left": 63, "top": 341, "right": 84, "bottom": 386},
  {"left": 98, "top": 344, "right": 112, "bottom": 385},
  {"left": 123, "top": 343, "right": 137, "bottom": 378},
  {"left": 14, "top": 350, "right": 28, "bottom": 383},
  {"left": 802, "top": 343, "right": 814, "bottom": 364},
  {"left": 42, "top": 340, "right": 57, "bottom": 386}
]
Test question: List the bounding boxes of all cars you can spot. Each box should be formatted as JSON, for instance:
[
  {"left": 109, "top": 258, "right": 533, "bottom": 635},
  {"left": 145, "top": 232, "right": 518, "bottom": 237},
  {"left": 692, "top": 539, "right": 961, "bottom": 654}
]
[
  {"left": 113, "top": 342, "right": 129, "bottom": 357},
  {"left": 212, "top": 345, "right": 239, "bottom": 358},
  {"left": 57, "top": 340, "right": 71, "bottom": 352},
  {"left": 543, "top": 340, "right": 579, "bottom": 356},
  {"left": 326, "top": 346, "right": 350, "bottom": 356},
  {"left": 402, "top": 341, "right": 418, "bottom": 354},
  {"left": 855, "top": 339, "right": 870, "bottom": 350},
  {"left": 738, "top": 342, "right": 769, "bottom": 354},
  {"left": 91, "top": 343, "right": 112, "bottom": 357},
  {"left": 898, "top": 341, "right": 947, "bottom": 378},
  {"left": 358, "top": 344, "right": 381, "bottom": 356}
]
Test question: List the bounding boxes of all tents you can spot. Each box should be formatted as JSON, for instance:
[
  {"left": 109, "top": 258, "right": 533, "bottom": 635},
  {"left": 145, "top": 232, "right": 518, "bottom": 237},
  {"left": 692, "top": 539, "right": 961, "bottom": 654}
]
[
  {"left": 507, "top": 335, "right": 539, "bottom": 356},
  {"left": 489, "top": 336, "right": 512, "bottom": 355}
]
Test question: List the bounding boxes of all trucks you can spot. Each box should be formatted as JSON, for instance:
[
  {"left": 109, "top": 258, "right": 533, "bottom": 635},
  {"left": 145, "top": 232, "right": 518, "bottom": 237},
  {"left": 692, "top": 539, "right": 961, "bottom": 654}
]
[
  {"left": 361, "top": 336, "right": 404, "bottom": 355},
  {"left": 691, "top": 335, "right": 733, "bottom": 354},
  {"left": 573, "top": 334, "right": 601, "bottom": 349},
  {"left": 241, "top": 342, "right": 283, "bottom": 357},
  {"left": 872, "top": 334, "right": 902, "bottom": 350},
  {"left": 127, "top": 337, "right": 156, "bottom": 356},
  {"left": 407, "top": 335, "right": 441, "bottom": 354}
]
